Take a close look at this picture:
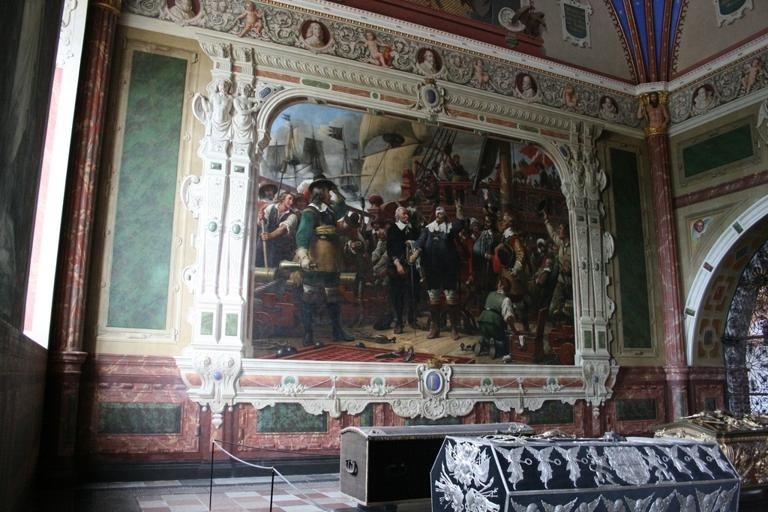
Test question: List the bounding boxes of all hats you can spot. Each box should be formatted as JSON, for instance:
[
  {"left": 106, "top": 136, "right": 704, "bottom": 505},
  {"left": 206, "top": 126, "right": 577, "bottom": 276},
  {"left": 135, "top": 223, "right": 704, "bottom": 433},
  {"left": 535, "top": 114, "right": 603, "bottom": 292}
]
[
  {"left": 370, "top": 217, "right": 385, "bottom": 229},
  {"left": 257, "top": 181, "right": 278, "bottom": 199},
  {"left": 344, "top": 212, "right": 359, "bottom": 228},
  {"left": 309, "top": 175, "right": 337, "bottom": 192}
]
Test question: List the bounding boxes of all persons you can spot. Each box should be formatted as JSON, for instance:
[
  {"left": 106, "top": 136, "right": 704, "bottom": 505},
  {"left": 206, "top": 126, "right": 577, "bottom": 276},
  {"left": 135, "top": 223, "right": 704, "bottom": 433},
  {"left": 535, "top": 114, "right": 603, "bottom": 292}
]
[
  {"left": 362, "top": 31, "right": 391, "bottom": 68},
  {"left": 420, "top": 51, "right": 439, "bottom": 74},
  {"left": 170, "top": 0, "right": 198, "bottom": 19},
  {"left": 235, "top": 0, "right": 263, "bottom": 37},
  {"left": 522, "top": 75, "right": 536, "bottom": 98},
  {"left": 602, "top": 97, "right": 617, "bottom": 118},
  {"left": 694, "top": 87, "right": 714, "bottom": 109},
  {"left": 474, "top": 58, "right": 490, "bottom": 87},
  {"left": 305, "top": 22, "right": 325, "bottom": 47},
  {"left": 741, "top": 57, "right": 763, "bottom": 94},
  {"left": 565, "top": 82, "right": 581, "bottom": 111},
  {"left": 637, "top": 92, "right": 669, "bottom": 130}
]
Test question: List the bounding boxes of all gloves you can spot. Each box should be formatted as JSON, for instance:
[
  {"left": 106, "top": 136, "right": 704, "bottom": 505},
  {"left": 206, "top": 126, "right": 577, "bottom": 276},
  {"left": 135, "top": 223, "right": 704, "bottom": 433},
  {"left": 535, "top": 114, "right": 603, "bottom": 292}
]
[{"left": 294, "top": 247, "right": 313, "bottom": 272}]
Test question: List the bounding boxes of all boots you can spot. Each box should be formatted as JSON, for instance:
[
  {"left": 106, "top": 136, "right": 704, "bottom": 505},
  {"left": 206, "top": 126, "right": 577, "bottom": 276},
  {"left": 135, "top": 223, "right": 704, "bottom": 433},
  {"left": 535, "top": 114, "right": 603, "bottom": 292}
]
[
  {"left": 489, "top": 338, "right": 504, "bottom": 360},
  {"left": 301, "top": 300, "right": 314, "bottom": 346},
  {"left": 325, "top": 302, "right": 354, "bottom": 342},
  {"left": 393, "top": 297, "right": 461, "bottom": 341},
  {"left": 474, "top": 336, "right": 489, "bottom": 357}
]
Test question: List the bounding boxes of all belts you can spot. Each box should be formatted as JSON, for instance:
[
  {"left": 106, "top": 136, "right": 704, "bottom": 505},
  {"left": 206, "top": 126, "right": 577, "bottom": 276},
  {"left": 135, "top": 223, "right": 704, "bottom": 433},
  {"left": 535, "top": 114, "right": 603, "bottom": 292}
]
[{"left": 483, "top": 307, "right": 501, "bottom": 316}]
